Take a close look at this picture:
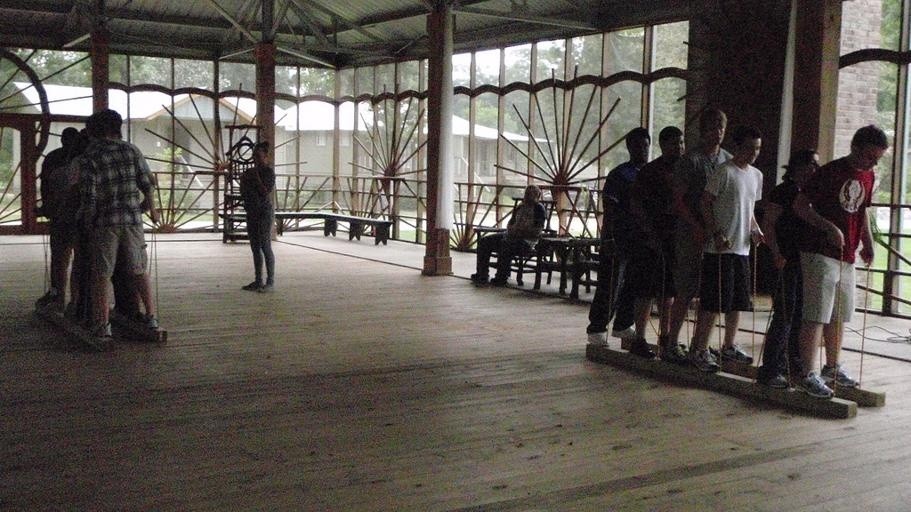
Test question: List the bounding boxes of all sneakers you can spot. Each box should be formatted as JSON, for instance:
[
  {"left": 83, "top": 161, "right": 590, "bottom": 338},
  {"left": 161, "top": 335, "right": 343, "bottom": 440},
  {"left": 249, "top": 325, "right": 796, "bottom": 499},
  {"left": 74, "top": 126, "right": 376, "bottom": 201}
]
[
  {"left": 709, "top": 344, "right": 721, "bottom": 357},
  {"left": 612, "top": 326, "right": 636, "bottom": 338},
  {"left": 242, "top": 281, "right": 265, "bottom": 291},
  {"left": 491, "top": 276, "right": 508, "bottom": 286},
  {"left": 721, "top": 344, "right": 754, "bottom": 365},
  {"left": 588, "top": 330, "right": 609, "bottom": 347},
  {"left": 658, "top": 335, "right": 687, "bottom": 349},
  {"left": 471, "top": 273, "right": 489, "bottom": 283},
  {"left": 36, "top": 291, "right": 158, "bottom": 336},
  {"left": 256, "top": 284, "right": 277, "bottom": 293},
  {"left": 796, "top": 373, "right": 835, "bottom": 399},
  {"left": 688, "top": 349, "right": 721, "bottom": 373},
  {"left": 630, "top": 337, "right": 657, "bottom": 358},
  {"left": 659, "top": 345, "right": 688, "bottom": 364},
  {"left": 822, "top": 365, "right": 861, "bottom": 386}
]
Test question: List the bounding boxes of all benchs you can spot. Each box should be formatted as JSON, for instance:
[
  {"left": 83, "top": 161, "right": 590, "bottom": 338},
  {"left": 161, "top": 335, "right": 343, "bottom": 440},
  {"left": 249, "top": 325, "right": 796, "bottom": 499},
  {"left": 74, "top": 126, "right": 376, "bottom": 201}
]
[
  {"left": 323, "top": 212, "right": 394, "bottom": 246},
  {"left": 218, "top": 207, "right": 326, "bottom": 236}
]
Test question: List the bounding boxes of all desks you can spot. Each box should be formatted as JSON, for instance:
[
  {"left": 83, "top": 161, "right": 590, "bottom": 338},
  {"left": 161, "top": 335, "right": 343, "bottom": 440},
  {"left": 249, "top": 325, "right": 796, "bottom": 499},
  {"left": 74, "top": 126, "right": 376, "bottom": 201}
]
[{"left": 532, "top": 233, "right": 592, "bottom": 296}]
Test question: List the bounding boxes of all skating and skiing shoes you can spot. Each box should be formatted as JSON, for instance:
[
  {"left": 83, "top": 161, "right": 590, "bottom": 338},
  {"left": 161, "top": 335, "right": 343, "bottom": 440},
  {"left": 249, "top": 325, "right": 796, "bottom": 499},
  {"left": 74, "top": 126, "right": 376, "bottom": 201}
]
[{"left": 757, "top": 368, "right": 790, "bottom": 388}]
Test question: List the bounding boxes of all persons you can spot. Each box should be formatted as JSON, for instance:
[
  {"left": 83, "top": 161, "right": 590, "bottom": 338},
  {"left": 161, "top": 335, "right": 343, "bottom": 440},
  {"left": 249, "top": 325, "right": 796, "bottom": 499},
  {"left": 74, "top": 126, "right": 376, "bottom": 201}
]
[
  {"left": 587, "top": 111, "right": 763, "bottom": 372},
  {"left": 757, "top": 126, "right": 889, "bottom": 398},
  {"left": 40, "top": 108, "right": 160, "bottom": 335},
  {"left": 241, "top": 142, "right": 276, "bottom": 292},
  {"left": 470, "top": 185, "right": 547, "bottom": 284}
]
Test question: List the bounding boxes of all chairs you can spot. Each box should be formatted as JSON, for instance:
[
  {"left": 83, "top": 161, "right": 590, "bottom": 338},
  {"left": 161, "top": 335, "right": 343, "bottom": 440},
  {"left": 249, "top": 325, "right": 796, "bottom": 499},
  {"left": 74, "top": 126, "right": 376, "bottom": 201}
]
[
  {"left": 473, "top": 195, "right": 561, "bottom": 288},
  {"left": 564, "top": 235, "right": 603, "bottom": 300}
]
